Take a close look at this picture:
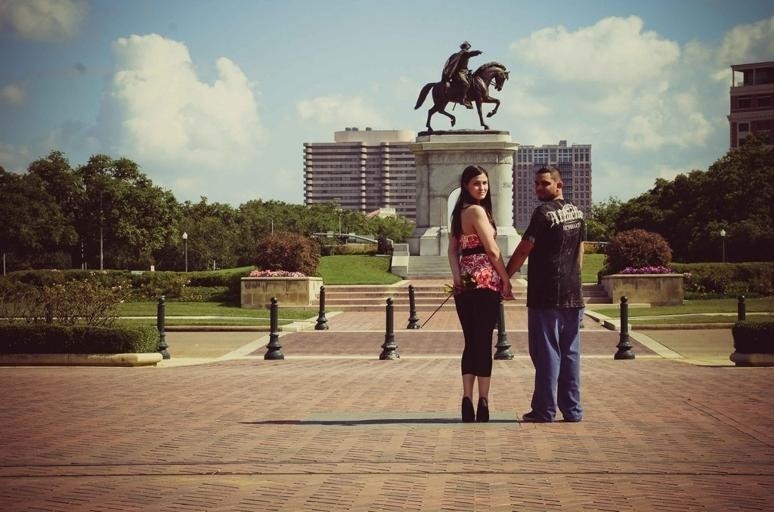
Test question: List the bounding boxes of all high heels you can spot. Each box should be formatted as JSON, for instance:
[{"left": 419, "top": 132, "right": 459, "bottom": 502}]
[
  {"left": 461, "top": 395, "right": 475, "bottom": 423},
  {"left": 475, "top": 396, "right": 490, "bottom": 423}
]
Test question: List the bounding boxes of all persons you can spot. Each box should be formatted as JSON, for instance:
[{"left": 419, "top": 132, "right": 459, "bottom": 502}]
[
  {"left": 497, "top": 166, "right": 588, "bottom": 423},
  {"left": 453, "top": 42, "right": 483, "bottom": 109},
  {"left": 446, "top": 165, "right": 513, "bottom": 422}
]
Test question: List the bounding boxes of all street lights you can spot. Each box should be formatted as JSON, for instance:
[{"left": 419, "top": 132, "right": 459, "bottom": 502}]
[
  {"left": 720, "top": 228, "right": 727, "bottom": 264},
  {"left": 182, "top": 232, "right": 189, "bottom": 273},
  {"left": 333, "top": 206, "right": 344, "bottom": 235},
  {"left": 99, "top": 187, "right": 111, "bottom": 271}
]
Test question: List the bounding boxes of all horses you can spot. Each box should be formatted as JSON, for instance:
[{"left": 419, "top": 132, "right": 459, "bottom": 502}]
[{"left": 414, "top": 60, "right": 510, "bottom": 132}]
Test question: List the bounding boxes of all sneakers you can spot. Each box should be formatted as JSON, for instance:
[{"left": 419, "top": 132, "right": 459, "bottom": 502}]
[{"left": 523, "top": 409, "right": 542, "bottom": 422}]
